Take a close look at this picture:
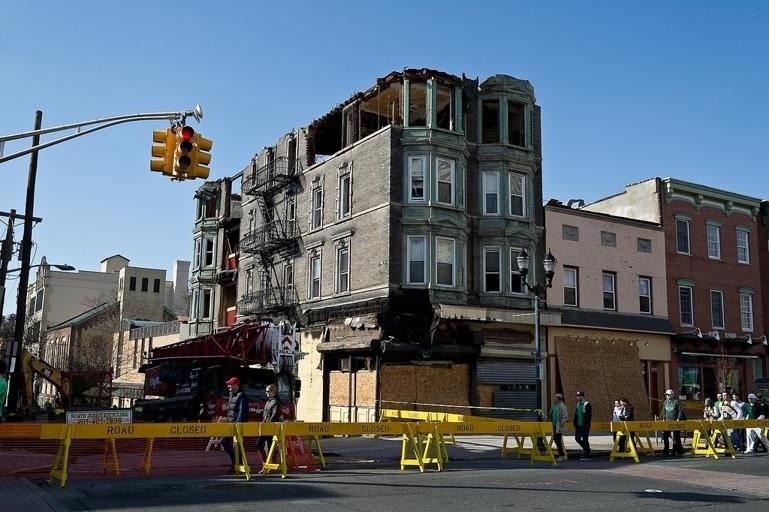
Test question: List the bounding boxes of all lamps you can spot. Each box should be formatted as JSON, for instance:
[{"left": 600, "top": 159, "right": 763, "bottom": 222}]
[
  {"left": 709, "top": 328, "right": 721, "bottom": 341},
  {"left": 689, "top": 328, "right": 702, "bottom": 339},
  {"left": 760, "top": 335, "right": 768, "bottom": 345},
  {"left": 742, "top": 333, "right": 753, "bottom": 344}
]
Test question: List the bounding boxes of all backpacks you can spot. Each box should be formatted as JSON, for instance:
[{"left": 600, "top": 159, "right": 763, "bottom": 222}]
[{"left": 756, "top": 399, "right": 769, "bottom": 417}]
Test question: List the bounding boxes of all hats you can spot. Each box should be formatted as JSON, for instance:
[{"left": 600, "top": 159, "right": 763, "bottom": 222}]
[
  {"left": 574, "top": 391, "right": 584, "bottom": 397},
  {"left": 665, "top": 389, "right": 673, "bottom": 395},
  {"left": 225, "top": 377, "right": 240, "bottom": 385},
  {"left": 748, "top": 393, "right": 757, "bottom": 399}
]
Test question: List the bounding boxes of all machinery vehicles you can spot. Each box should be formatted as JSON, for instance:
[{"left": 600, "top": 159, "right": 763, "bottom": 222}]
[
  {"left": 6, "top": 348, "right": 113, "bottom": 422},
  {"left": 132, "top": 319, "right": 310, "bottom": 447}
]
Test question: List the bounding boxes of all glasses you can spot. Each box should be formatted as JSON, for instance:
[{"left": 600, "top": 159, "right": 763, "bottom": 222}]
[{"left": 265, "top": 390, "right": 270, "bottom": 393}]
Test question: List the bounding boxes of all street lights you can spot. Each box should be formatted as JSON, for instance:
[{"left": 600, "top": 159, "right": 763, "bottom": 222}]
[
  {"left": 1, "top": 265, "right": 75, "bottom": 319},
  {"left": 515, "top": 247, "right": 556, "bottom": 453}
]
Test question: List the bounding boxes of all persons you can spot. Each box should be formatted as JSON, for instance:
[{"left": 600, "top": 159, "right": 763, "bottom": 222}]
[
  {"left": 573, "top": 390, "right": 593, "bottom": 461},
  {"left": 703, "top": 391, "right": 769, "bottom": 454},
  {"left": 221, "top": 376, "right": 249, "bottom": 475},
  {"left": 610, "top": 397, "right": 637, "bottom": 461},
  {"left": 657, "top": 389, "right": 686, "bottom": 457},
  {"left": 548, "top": 394, "right": 569, "bottom": 464},
  {"left": 255, "top": 385, "right": 281, "bottom": 474}
]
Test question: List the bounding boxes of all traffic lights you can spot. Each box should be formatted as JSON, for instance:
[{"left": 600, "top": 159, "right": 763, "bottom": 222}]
[
  {"left": 171, "top": 126, "right": 201, "bottom": 177},
  {"left": 196, "top": 131, "right": 214, "bottom": 178},
  {"left": 149, "top": 129, "right": 175, "bottom": 176}
]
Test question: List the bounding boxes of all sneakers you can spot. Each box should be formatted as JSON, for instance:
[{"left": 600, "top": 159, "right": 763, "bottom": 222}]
[
  {"left": 579, "top": 454, "right": 592, "bottom": 461},
  {"left": 556, "top": 455, "right": 568, "bottom": 462}
]
